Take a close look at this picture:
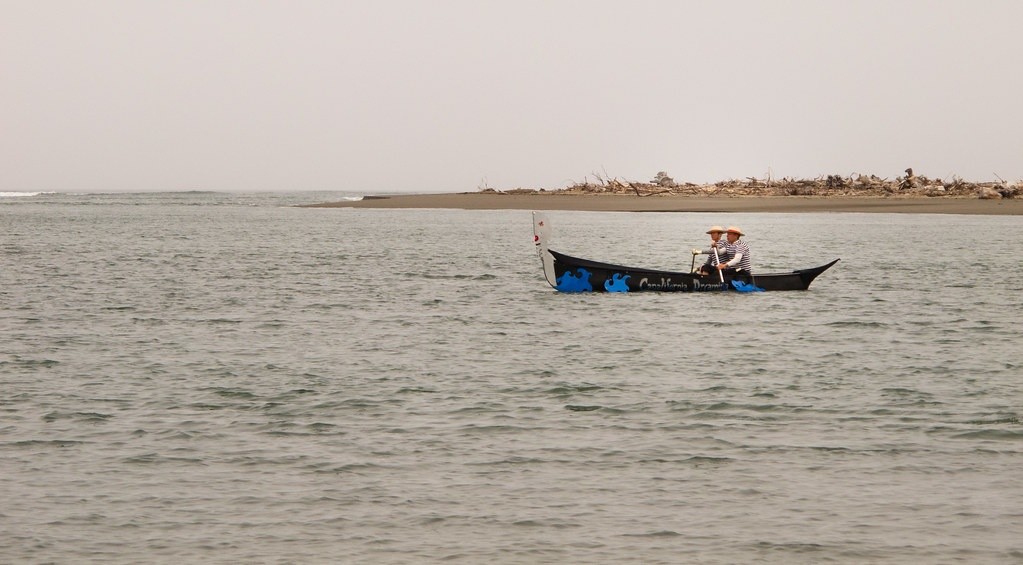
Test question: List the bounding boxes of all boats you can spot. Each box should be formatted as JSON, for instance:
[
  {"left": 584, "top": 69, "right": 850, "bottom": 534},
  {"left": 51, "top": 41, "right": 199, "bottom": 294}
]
[{"left": 530, "top": 211, "right": 841, "bottom": 294}]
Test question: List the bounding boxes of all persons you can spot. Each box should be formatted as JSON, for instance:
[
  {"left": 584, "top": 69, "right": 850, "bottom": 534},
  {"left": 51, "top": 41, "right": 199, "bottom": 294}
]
[
  {"left": 692, "top": 226, "right": 728, "bottom": 274},
  {"left": 711, "top": 227, "right": 751, "bottom": 274}
]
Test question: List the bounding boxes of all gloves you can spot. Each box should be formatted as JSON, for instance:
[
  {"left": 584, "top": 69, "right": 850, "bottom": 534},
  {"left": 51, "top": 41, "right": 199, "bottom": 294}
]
[
  {"left": 692, "top": 268, "right": 698, "bottom": 273},
  {"left": 692, "top": 249, "right": 702, "bottom": 254}
]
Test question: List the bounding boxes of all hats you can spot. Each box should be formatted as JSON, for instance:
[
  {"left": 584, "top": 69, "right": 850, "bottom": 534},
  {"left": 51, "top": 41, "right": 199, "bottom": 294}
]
[
  {"left": 706, "top": 226, "right": 726, "bottom": 234},
  {"left": 723, "top": 226, "right": 745, "bottom": 236}
]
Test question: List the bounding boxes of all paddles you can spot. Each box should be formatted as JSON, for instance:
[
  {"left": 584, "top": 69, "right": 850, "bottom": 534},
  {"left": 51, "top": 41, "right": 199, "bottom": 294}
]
[
  {"left": 713, "top": 246, "right": 729, "bottom": 291},
  {"left": 691, "top": 254, "right": 696, "bottom": 274}
]
